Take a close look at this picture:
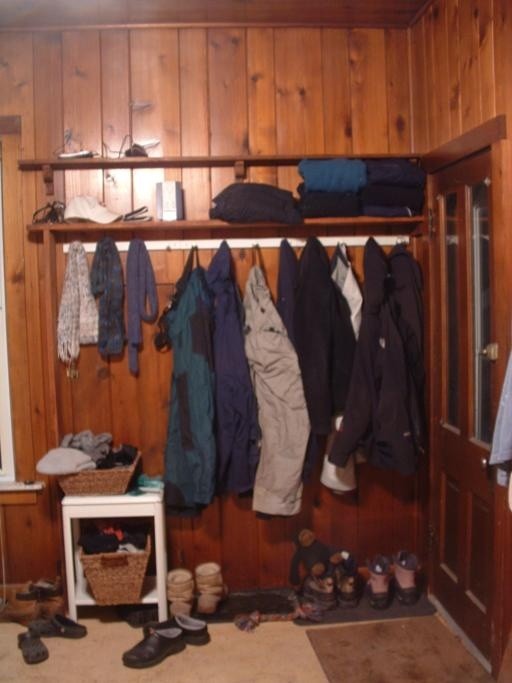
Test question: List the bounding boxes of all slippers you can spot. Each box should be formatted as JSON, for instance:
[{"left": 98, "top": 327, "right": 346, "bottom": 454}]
[
  {"left": 16, "top": 631, "right": 52, "bottom": 667},
  {"left": 26, "top": 613, "right": 88, "bottom": 640}
]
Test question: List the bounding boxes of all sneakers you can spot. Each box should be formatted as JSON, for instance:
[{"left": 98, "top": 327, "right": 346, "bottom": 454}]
[
  {"left": 152, "top": 614, "right": 218, "bottom": 646},
  {"left": 122, "top": 625, "right": 189, "bottom": 670},
  {"left": 336, "top": 571, "right": 363, "bottom": 607},
  {"left": 302, "top": 571, "right": 339, "bottom": 610}
]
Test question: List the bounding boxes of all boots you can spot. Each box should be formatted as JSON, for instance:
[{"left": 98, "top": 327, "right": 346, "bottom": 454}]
[
  {"left": 194, "top": 560, "right": 233, "bottom": 615},
  {"left": 390, "top": 548, "right": 420, "bottom": 606},
  {"left": 365, "top": 553, "right": 391, "bottom": 612},
  {"left": 166, "top": 568, "right": 196, "bottom": 619}
]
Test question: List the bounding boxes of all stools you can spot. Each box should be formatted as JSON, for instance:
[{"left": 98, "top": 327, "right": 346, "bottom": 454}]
[{"left": 61, "top": 481, "right": 168, "bottom": 624}]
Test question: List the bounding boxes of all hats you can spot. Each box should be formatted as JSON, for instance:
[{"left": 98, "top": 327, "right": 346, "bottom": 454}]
[{"left": 61, "top": 192, "right": 122, "bottom": 225}]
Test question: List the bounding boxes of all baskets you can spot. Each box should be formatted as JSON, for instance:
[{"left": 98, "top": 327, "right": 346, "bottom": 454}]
[
  {"left": 79, "top": 533, "right": 152, "bottom": 607},
  {"left": 56, "top": 449, "right": 144, "bottom": 497}
]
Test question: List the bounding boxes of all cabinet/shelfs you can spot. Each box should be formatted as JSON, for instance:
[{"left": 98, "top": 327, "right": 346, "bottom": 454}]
[{"left": 17, "top": 152, "right": 436, "bottom": 231}]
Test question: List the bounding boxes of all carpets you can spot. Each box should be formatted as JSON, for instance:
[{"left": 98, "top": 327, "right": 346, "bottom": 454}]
[{"left": 306, "top": 615, "right": 493, "bottom": 683}]
